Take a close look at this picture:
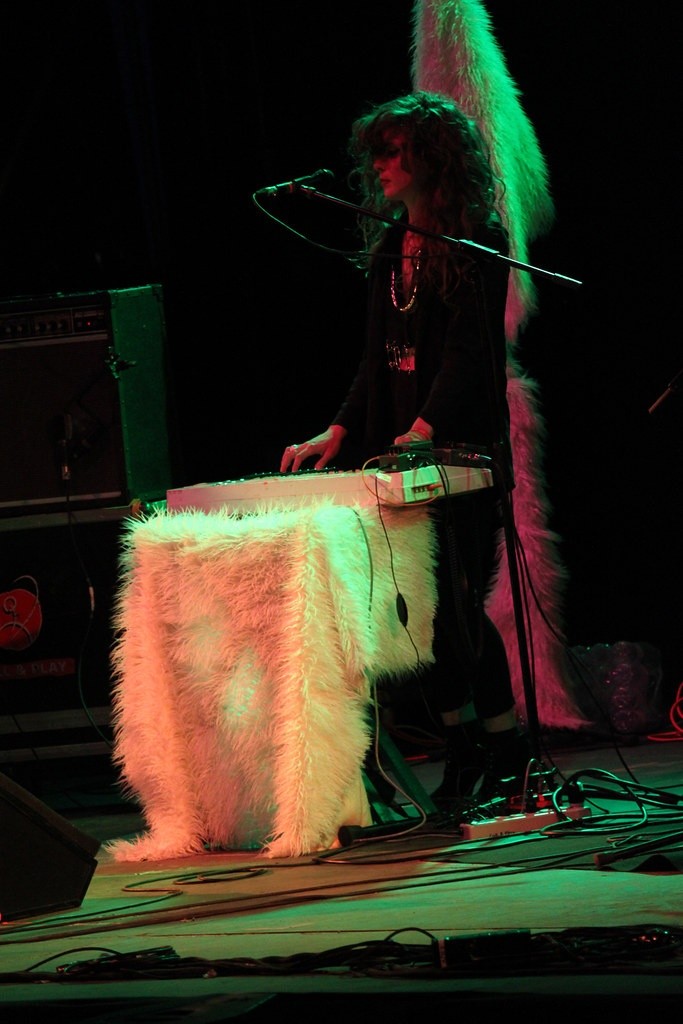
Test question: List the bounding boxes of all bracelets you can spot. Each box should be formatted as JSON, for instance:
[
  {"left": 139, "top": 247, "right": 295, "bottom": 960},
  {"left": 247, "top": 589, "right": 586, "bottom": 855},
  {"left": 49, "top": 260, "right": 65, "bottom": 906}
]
[{"left": 405, "top": 431, "right": 427, "bottom": 441}]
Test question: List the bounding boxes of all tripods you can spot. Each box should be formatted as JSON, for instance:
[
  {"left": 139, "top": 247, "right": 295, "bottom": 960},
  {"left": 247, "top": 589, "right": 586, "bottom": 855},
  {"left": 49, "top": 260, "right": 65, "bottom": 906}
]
[{"left": 300, "top": 181, "right": 683, "bottom": 849}]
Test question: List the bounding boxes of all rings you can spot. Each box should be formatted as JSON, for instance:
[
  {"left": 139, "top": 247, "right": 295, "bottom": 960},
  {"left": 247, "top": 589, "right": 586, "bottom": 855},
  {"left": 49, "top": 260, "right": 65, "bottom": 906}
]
[{"left": 290, "top": 444, "right": 299, "bottom": 455}]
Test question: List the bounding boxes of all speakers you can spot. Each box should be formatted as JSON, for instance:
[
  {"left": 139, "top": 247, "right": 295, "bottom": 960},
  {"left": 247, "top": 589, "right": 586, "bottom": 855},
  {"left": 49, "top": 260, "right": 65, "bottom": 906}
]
[
  {"left": 0, "top": 507, "right": 146, "bottom": 757},
  {"left": 0, "top": 282, "right": 172, "bottom": 512}
]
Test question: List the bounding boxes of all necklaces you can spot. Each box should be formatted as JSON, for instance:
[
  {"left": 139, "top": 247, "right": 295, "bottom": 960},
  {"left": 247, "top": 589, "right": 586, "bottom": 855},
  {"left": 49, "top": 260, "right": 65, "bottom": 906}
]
[{"left": 384, "top": 249, "right": 422, "bottom": 375}]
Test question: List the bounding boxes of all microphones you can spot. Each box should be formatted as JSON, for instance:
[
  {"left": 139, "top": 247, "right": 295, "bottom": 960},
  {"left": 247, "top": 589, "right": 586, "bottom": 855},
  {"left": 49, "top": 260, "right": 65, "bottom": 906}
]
[{"left": 256, "top": 169, "right": 334, "bottom": 197}]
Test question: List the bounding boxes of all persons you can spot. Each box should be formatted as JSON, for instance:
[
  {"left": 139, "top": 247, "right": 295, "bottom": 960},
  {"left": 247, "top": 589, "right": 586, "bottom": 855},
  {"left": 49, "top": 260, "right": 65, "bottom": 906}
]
[{"left": 280, "top": 90, "right": 534, "bottom": 806}]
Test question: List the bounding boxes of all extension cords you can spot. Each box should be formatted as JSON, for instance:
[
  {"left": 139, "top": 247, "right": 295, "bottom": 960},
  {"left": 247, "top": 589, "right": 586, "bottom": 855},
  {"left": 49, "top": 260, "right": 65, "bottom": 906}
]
[{"left": 460, "top": 803, "right": 595, "bottom": 839}]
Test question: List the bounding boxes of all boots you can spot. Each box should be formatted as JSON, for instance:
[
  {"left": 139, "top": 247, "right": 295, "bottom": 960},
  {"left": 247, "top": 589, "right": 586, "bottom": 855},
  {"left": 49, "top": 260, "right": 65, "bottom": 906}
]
[
  {"left": 430, "top": 719, "right": 484, "bottom": 810},
  {"left": 473, "top": 727, "right": 524, "bottom": 804}
]
